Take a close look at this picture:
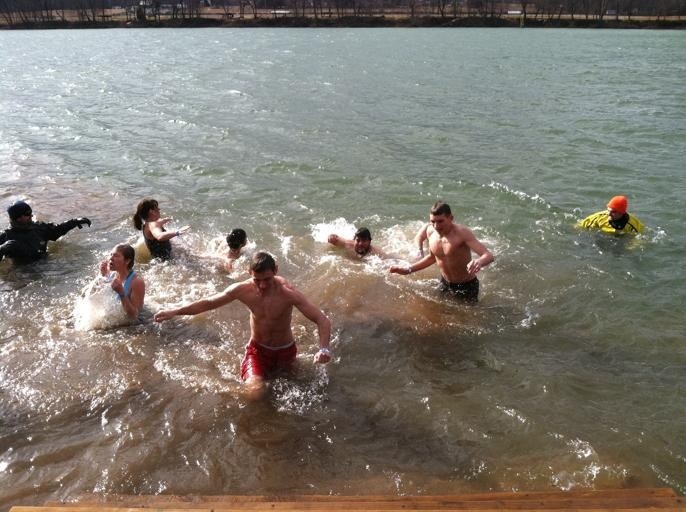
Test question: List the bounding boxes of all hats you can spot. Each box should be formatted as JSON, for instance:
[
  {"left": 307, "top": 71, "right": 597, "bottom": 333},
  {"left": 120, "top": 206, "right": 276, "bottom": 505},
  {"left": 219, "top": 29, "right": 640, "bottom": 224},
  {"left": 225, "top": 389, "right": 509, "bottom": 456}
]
[
  {"left": 6, "top": 200, "right": 33, "bottom": 221},
  {"left": 354, "top": 226, "right": 372, "bottom": 240},
  {"left": 605, "top": 195, "right": 628, "bottom": 215}
]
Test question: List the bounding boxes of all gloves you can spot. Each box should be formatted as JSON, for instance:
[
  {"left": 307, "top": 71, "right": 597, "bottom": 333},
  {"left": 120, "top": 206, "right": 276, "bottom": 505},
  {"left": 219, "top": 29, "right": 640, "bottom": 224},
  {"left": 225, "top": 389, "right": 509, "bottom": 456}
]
[
  {"left": 75, "top": 216, "right": 92, "bottom": 230},
  {"left": 0, "top": 239, "right": 19, "bottom": 256}
]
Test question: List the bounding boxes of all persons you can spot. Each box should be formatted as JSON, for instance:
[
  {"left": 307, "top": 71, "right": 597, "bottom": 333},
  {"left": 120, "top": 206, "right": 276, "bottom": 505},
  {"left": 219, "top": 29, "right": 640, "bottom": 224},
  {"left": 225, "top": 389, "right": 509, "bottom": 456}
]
[
  {"left": 153, "top": 250, "right": 332, "bottom": 404},
  {"left": 0, "top": 200, "right": 92, "bottom": 268},
  {"left": 570, "top": 195, "right": 650, "bottom": 253},
  {"left": 223, "top": 227, "right": 249, "bottom": 261},
  {"left": 132, "top": 196, "right": 193, "bottom": 262},
  {"left": 389, "top": 200, "right": 498, "bottom": 302},
  {"left": 412, "top": 224, "right": 436, "bottom": 256},
  {"left": 100, "top": 244, "right": 146, "bottom": 321},
  {"left": 326, "top": 227, "right": 386, "bottom": 259}
]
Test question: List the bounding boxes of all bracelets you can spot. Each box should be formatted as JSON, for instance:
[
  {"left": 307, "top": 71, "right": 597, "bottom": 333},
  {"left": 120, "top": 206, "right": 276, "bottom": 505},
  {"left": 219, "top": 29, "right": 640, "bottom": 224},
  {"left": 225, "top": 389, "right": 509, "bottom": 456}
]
[
  {"left": 176, "top": 230, "right": 180, "bottom": 237},
  {"left": 119, "top": 292, "right": 125, "bottom": 298},
  {"left": 407, "top": 262, "right": 413, "bottom": 274},
  {"left": 318, "top": 347, "right": 331, "bottom": 354}
]
